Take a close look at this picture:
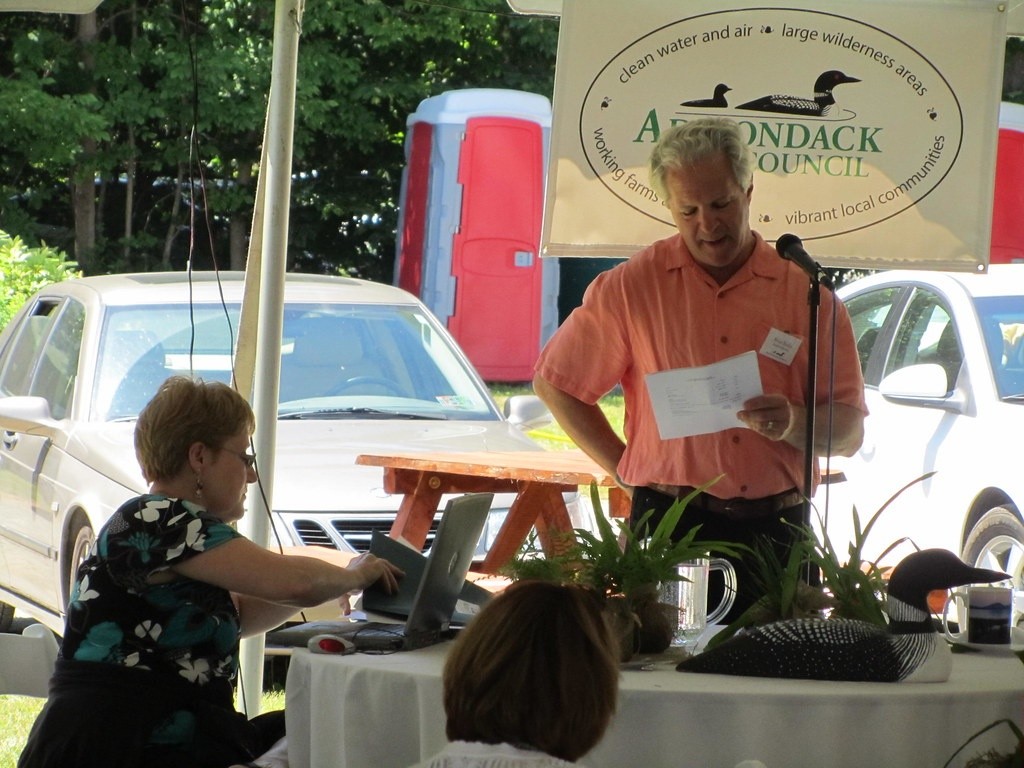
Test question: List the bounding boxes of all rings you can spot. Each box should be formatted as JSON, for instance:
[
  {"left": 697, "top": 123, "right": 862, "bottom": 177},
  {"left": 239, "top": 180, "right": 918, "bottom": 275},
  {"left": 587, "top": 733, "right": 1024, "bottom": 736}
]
[{"left": 767, "top": 421, "right": 773, "bottom": 430}]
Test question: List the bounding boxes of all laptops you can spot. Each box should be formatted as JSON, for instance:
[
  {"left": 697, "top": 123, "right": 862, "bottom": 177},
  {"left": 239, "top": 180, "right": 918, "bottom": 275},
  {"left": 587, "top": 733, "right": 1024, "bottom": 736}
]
[{"left": 271, "top": 492, "right": 498, "bottom": 652}]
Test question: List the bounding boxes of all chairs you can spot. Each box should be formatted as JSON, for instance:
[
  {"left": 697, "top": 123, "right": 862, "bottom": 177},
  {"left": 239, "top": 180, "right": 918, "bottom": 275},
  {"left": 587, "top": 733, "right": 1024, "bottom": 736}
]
[
  {"left": 289, "top": 319, "right": 385, "bottom": 399},
  {"left": 934, "top": 311, "right": 1004, "bottom": 396},
  {"left": 105, "top": 333, "right": 167, "bottom": 420}
]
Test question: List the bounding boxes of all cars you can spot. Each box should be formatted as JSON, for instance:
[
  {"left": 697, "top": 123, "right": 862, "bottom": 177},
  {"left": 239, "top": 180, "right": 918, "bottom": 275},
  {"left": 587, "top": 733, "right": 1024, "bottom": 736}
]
[
  {"left": 0, "top": 271, "right": 579, "bottom": 653},
  {"left": 804, "top": 264, "right": 1024, "bottom": 634}
]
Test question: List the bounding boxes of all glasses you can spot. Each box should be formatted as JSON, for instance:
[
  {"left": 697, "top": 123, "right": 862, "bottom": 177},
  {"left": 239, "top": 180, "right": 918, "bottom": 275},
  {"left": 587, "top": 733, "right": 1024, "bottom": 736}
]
[{"left": 214, "top": 443, "right": 256, "bottom": 467}]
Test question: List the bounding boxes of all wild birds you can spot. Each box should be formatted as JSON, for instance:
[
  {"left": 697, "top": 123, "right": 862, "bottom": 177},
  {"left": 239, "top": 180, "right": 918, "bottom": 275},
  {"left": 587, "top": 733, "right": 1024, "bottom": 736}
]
[
  {"left": 677, "top": 549, "right": 1012, "bottom": 686},
  {"left": 736, "top": 70, "right": 862, "bottom": 118},
  {"left": 681, "top": 84, "right": 732, "bottom": 109}
]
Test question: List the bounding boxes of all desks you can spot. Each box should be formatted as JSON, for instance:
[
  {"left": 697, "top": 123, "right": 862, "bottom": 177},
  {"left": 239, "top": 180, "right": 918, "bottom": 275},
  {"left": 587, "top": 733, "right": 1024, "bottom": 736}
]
[
  {"left": 353, "top": 446, "right": 630, "bottom": 576},
  {"left": 285, "top": 626, "right": 1024, "bottom": 768}
]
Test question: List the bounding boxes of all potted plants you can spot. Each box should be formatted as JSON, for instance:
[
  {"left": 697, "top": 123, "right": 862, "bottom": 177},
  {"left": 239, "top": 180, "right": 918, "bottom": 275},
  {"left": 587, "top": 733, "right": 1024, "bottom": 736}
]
[{"left": 493, "top": 471, "right": 756, "bottom": 656}]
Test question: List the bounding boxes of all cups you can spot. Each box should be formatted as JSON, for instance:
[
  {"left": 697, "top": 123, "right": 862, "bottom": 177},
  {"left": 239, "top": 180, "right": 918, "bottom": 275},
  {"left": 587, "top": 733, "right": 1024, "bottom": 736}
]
[
  {"left": 655, "top": 546, "right": 738, "bottom": 646},
  {"left": 942, "top": 585, "right": 1013, "bottom": 645}
]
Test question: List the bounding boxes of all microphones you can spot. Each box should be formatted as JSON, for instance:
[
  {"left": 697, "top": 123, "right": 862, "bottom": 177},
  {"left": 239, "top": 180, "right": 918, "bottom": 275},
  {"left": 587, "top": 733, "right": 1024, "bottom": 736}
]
[{"left": 777, "top": 233, "right": 836, "bottom": 291}]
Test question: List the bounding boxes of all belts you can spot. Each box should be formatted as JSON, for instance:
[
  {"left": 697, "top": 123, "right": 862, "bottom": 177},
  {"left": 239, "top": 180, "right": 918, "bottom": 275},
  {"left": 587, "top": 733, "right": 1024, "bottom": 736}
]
[{"left": 645, "top": 482, "right": 804, "bottom": 520}]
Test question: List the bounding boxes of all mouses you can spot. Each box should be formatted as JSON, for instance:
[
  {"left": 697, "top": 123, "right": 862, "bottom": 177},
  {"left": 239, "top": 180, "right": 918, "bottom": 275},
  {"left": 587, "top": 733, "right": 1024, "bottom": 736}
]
[{"left": 307, "top": 633, "right": 355, "bottom": 654}]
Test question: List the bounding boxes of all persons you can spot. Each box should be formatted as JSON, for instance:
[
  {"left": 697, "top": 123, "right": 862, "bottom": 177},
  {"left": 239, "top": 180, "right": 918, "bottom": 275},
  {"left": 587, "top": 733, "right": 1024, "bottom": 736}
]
[
  {"left": 18, "top": 377, "right": 406, "bottom": 768},
  {"left": 533, "top": 119, "right": 870, "bottom": 625},
  {"left": 414, "top": 581, "right": 620, "bottom": 768}
]
